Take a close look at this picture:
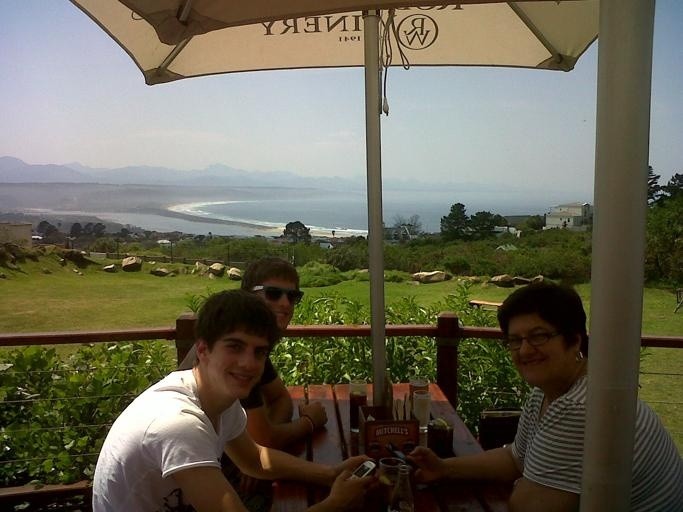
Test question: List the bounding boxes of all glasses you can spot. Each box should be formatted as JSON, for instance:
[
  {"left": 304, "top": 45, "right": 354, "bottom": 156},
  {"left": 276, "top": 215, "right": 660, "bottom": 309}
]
[
  {"left": 502, "top": 330, "right": 565, "bottom": 351},
  {"left": 253, "top": 284, "right": 304, "bottom": 306}
]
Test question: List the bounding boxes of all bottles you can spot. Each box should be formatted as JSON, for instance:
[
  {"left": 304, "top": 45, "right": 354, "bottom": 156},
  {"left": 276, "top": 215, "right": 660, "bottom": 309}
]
[
  {"left": 385, "top": 465, "right": 415, "bottom": 512},
  {"left": 384, "top": 368, "right": 394, "bottom": 409}
]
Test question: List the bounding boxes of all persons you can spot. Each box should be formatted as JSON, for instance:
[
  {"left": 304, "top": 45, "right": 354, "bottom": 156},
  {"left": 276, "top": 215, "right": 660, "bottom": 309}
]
[
  {"left": 404, "top": 280, "right": 683, "bottom": 512},
  {"left": 178, "top": 256, "right": 329, "bottom": 512},
  {"left": 91, "top": 288, "right": 378, "bottom": 512}
]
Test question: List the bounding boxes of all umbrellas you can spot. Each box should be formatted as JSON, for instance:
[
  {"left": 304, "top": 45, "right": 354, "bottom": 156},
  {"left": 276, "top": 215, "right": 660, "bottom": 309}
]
[{"left": 68, "top": 0, "right": 600, "bottom": 423}]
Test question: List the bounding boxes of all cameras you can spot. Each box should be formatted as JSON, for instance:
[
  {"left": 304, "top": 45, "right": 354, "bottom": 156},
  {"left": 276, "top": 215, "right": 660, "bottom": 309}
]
[{"left": 427, "top": 418, "right": 454, "bottom": 456}]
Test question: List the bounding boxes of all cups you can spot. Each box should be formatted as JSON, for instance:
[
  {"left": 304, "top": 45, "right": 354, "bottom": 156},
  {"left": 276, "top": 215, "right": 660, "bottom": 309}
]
[
  {"left": 378, "top": 457, "right": 406, "bottom": 506},
  {"left": 408, "top": 376, "right": 431, "bottom": 435},
  {"left": 427, "top": 420, "right": 455, "bottom": 459},
  {"left": 348, "top": 379, "right": 368, "bottom": 434}
]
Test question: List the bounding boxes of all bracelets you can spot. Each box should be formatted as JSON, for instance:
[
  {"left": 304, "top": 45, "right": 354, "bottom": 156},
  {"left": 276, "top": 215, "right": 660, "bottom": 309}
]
[{"left": 303, "top": 414, "right": 316, "bottom": 432}]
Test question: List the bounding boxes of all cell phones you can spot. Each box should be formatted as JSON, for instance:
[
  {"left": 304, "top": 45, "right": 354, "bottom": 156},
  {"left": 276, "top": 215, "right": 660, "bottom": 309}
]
[
  {"left": 304, "top": 382, "right": 309, "bottom": 404},
  {"left": 347, "top": 459, "right": 376, "bottom": 483},
  {"left": 384, "top": 438, "right": 419, "bottom": 471}
]
